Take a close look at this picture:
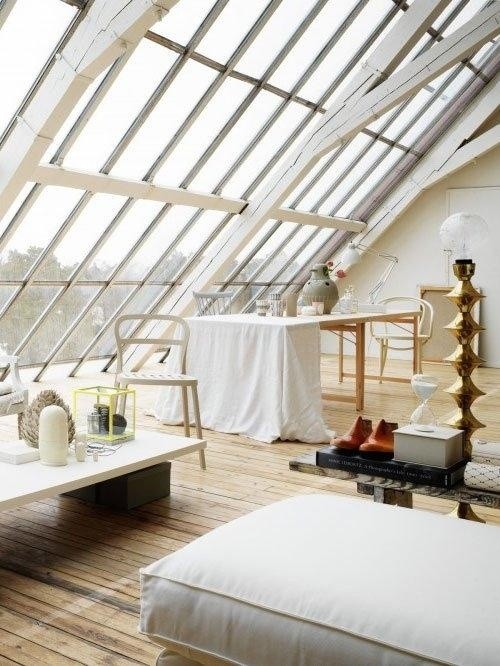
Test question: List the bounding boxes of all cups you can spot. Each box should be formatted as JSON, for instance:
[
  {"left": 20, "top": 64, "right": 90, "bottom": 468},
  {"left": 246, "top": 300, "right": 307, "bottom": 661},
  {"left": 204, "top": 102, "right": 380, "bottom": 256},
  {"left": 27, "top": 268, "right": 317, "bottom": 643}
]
[{"left": 254, "top": 284, "right": 358, "bottom": 317}]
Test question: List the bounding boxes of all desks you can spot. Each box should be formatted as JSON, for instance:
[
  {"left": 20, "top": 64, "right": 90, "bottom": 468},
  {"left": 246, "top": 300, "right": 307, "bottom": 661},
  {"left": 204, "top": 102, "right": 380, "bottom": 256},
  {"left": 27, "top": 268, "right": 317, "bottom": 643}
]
[
  {"left": 288, "top": 449, "right": 500, "bottom": 510},
  {"left": 0, "top": 424, "right": 207, "bottom": 513},
  {"left": 416, "top": 285, "right": 481, "bottom": 367}
]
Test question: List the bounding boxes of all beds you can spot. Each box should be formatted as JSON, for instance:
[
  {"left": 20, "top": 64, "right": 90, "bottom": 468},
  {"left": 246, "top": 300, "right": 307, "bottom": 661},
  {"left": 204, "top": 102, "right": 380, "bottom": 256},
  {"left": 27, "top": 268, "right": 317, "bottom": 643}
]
[{"left": 137, "top": 490, "right": 500, "bottom": 666}]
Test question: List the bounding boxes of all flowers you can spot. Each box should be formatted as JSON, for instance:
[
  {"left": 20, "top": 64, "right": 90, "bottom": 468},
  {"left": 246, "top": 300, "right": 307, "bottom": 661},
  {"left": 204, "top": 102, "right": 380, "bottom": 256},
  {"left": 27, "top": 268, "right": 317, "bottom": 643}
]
[{"left": 324, "top": 261, "right": 348, "bottom": 278}]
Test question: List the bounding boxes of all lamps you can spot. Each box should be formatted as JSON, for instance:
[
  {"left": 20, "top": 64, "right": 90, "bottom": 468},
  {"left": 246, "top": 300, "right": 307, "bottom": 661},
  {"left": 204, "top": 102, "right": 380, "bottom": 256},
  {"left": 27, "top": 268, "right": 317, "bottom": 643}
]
[
  {"left": 348, "top": 240, "right": 399, "bottom": 304},
  {"left": 439, "top": 213, "right": 487, "bottom": 523}
]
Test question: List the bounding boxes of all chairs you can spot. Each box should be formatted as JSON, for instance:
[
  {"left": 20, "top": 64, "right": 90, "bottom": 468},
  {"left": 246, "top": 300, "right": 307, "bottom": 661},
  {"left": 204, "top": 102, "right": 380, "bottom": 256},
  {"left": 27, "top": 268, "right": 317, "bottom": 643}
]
[
  {"left": 111, "top": 313, "right": 208, "bottom": 471},
  {"left": 191, "top": 291, "right": 233, "bottom": 316},
  {"left": 370, "top": 296, "right": 434, "bottom": 384},
  {"left": 144, "top": 308, "right": 422, "bottom": 445},
  {"left": 0, "top": 351, "right": 29, "bottom": 441}
]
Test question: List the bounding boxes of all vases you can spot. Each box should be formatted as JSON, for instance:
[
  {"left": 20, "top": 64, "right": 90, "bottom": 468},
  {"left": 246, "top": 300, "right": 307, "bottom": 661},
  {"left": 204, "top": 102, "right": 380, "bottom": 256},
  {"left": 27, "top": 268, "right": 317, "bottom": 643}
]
[{"left": 303, "top": 264, "right": 338, "bottom": 314}]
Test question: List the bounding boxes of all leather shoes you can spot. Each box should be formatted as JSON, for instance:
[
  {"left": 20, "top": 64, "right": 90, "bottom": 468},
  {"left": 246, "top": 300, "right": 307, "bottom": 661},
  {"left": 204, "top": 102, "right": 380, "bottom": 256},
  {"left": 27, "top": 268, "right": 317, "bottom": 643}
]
[
  {"left": 358, "top": 418, "right": 398, "bottom": 458},
  {"left": 329, "top": 415, "right": 372, "bottom": 454}
]
